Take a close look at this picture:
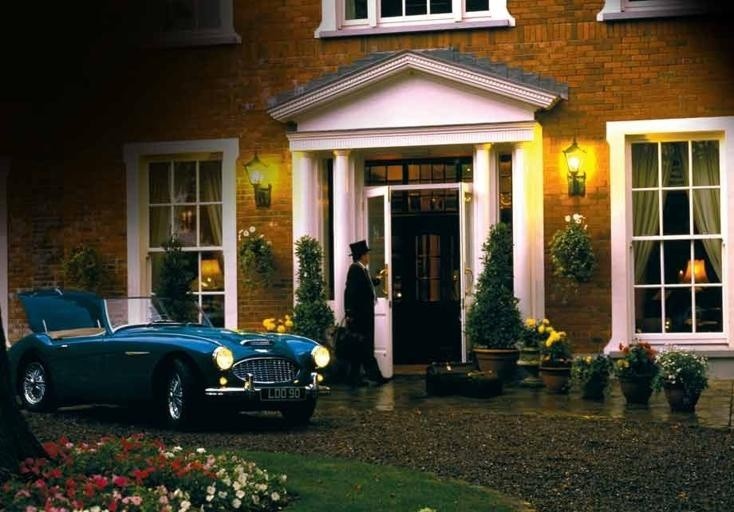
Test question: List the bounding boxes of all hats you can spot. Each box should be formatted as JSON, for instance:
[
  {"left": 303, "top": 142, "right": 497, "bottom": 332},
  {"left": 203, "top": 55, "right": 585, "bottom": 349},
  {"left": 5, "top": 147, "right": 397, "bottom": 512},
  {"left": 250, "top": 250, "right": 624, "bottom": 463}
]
[{"left": 347, "top": 240, "right": 372, "bottom": 257}]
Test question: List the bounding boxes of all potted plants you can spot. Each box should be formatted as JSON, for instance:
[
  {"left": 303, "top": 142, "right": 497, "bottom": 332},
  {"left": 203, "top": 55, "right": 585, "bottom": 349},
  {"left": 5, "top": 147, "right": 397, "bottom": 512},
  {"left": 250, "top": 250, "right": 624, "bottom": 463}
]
[
  {"left": 291, "top": 234, "right": 335, "bottom": 384},
  {"left": 464, "top": 222, "right": 525, "bottom": 379}
]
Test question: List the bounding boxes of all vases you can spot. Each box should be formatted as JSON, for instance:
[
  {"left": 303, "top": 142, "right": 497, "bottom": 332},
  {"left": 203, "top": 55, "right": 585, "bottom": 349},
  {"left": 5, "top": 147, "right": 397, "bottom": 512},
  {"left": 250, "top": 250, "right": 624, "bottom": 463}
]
[
  {"left": 619, "top": 374, "right": 653, "bottom": 409},
  {"left": 660, "top": 380, "right": 700, "bottom": 413},
  {"left": 540, "top": 362, "right": 572, "bottom": 395},
  {"left": 583, "top": 376, "right": 603, "bottom": 402}
]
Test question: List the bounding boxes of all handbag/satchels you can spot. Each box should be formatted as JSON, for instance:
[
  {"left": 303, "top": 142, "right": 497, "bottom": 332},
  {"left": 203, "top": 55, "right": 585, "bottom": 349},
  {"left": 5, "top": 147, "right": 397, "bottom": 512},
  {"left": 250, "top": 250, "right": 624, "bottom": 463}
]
[{"left": 333, "top": 315, "right": 371, "bottom": 364}]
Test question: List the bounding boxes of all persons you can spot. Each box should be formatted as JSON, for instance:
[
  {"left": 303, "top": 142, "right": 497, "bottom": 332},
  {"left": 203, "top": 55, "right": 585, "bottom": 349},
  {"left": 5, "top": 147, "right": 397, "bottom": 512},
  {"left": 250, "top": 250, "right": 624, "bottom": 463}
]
[{"left": 343, "top": 239, "right": 389, "bottom": 386}]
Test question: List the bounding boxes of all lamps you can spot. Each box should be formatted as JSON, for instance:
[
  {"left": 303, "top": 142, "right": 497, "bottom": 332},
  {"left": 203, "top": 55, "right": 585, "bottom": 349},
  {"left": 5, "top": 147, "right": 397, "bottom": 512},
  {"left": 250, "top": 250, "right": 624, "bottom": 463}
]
[
  {"left": 200, "top": 257, "right": 222, "bottom": 306},
  {"left": 243, "top": 151, "right": 273, "bottom": 208},
  {"left": 680, "top": 259, "right": 708, "bottom": 311},
  {"left": 561, "top": 134, "right": 588, "bottom": 196}
]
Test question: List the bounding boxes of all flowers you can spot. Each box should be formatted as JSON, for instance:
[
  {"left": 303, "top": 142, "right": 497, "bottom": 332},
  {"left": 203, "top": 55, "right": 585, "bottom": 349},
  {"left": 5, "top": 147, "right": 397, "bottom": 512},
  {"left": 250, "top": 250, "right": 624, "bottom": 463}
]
[
  {"left": 577, "top": 353, "right": 609, "bottom": 378},
  {"left": 525, "top": 314, "right": 569, "bottom": 367},
  {"left": 261, "top": 315, "right": 293, "bottom": 334},
  {"left": 237, "top": 225, "right": 272, "bottom": 277},
  {"left": 616, "top": 337, "right": 660, "bottom": 374},
  {"left": 654, "top": 343, "right": 707, "bottom": 383},
  {"left": 547, "top": 212, "right": 596, "bottom": 285}
]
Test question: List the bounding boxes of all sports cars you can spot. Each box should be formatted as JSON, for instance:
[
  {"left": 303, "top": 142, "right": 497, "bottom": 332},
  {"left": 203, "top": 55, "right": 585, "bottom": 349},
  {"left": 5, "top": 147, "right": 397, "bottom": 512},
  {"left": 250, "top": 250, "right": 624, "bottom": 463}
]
[{"left": 7, "top": 289, "right": 334, "bottom": 435}]
